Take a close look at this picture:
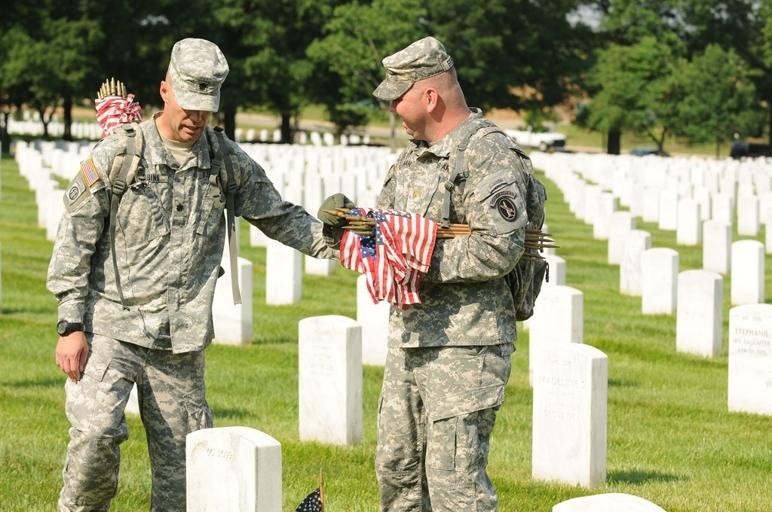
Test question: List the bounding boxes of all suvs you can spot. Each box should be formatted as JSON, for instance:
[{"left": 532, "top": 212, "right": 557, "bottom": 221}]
[{"left": 730, "top": 143, "right": 771, "bottom": 161}]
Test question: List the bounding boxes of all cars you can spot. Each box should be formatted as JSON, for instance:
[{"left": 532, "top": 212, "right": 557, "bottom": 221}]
[{"left": 630, "top": 149, "right": 670, "bottom": 156}]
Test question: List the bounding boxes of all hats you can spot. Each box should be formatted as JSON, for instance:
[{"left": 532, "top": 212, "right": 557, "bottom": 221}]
[
  {"left": 373, "top": 37, "right": 455, "bottom": 102},
  {"left": 170, "top": 38, "right": 229, "bottom": 112}
]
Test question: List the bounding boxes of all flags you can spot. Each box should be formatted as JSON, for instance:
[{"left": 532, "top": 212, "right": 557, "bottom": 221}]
[{"left": 338, "top": 204, "right": 439, "bottom": 312}]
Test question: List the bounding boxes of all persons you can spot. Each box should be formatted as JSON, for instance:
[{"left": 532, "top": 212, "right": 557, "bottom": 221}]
[
  {"left": 46, "top": 39, "right": 340, "bottom": 511},
  {"left": 322, "top": 37, "right": 550, "bottom": 512}
]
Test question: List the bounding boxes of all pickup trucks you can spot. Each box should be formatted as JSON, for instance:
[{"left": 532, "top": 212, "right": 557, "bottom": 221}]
[{"left": 505, "top": 126, "right": 566, "bottom": 152}]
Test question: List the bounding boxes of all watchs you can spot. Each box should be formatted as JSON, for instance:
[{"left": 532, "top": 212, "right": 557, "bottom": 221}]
[{"left": 56, "top": 318, "right": 84, "bottom": 335}]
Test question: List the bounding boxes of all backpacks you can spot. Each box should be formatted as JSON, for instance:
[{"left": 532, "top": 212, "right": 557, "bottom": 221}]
[{"left": 444, "top": 117, "right": 548, "bottom": 321}]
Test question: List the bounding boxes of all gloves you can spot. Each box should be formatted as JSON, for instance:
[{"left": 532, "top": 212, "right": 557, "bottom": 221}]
[{"left": 318, "top": 194, "right": 356, "bottom": 228}]
